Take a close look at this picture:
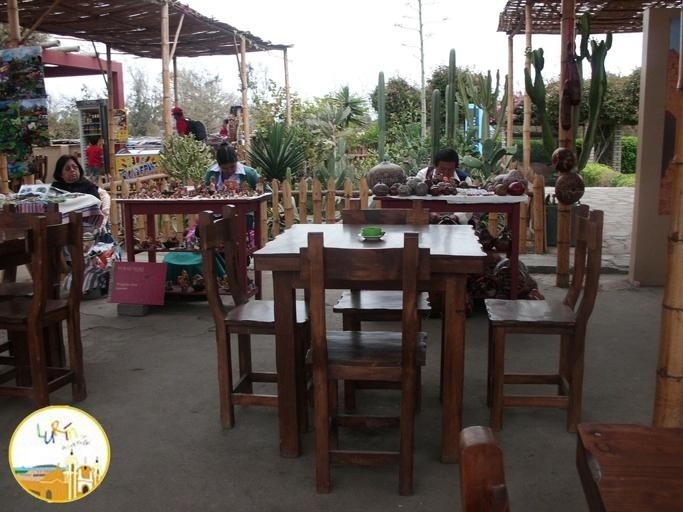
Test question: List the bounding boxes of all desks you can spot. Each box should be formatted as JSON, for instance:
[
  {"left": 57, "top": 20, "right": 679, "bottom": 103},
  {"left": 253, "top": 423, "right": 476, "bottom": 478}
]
[
  {"left": 573, "top": 421, "right": 683, "bottom": 511},
  {"left": 110, "top": 192, "right": 275, "bottom": 303}
]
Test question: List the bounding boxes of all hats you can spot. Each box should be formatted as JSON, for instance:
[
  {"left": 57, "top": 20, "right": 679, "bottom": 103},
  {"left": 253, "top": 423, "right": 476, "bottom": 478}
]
[{"left": 171, "top": 108, "right": 183, "bottom": 115}]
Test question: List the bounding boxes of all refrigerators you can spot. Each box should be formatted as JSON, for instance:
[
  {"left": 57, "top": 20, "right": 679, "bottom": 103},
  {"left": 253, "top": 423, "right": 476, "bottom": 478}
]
[
  {"left": 114, "top": 146, "right": 163, "bottom": 179},
  {"left": 76, "top": 98, "right": 110, "bottom": 181}
]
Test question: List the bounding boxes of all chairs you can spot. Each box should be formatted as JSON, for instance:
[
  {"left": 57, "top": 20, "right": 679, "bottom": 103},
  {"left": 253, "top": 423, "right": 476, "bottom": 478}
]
[
  {"left": 196, "top": 196, "right": 607, "bottom": 498},
  {"left": 0, "top": 198, "right": 88, "bottom": 415}
]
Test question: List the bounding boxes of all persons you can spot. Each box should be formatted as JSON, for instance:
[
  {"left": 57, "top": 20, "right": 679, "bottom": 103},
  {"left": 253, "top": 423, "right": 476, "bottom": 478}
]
[
  {"left": 170, "top": 107, "right": 190, "bottom": 137},
  {"left": 202, "top": 141, "right": 261, "bottom": 232},
  {"left": 50, "top": 154, "right": 102, "bottom": 278},
  {"left": 218, "top": 119, "right": 228, "bottom": 137},
  {"left": 84, "top": 138, "right": 103, "bottom": 179},
  {"left": 413, "top": 147, "right": 474, "bottom": 225}
]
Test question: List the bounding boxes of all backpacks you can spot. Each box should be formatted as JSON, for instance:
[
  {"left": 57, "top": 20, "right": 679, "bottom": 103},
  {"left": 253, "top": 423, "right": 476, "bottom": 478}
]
[{"left": 186, "top": 119, "right": 207, "bottom": 140}]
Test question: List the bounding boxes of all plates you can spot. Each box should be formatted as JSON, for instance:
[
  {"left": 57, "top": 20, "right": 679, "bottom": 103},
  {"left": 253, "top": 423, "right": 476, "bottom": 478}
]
[{"left": 359, "top": 231, "right": 385, "bottom": 241}]
